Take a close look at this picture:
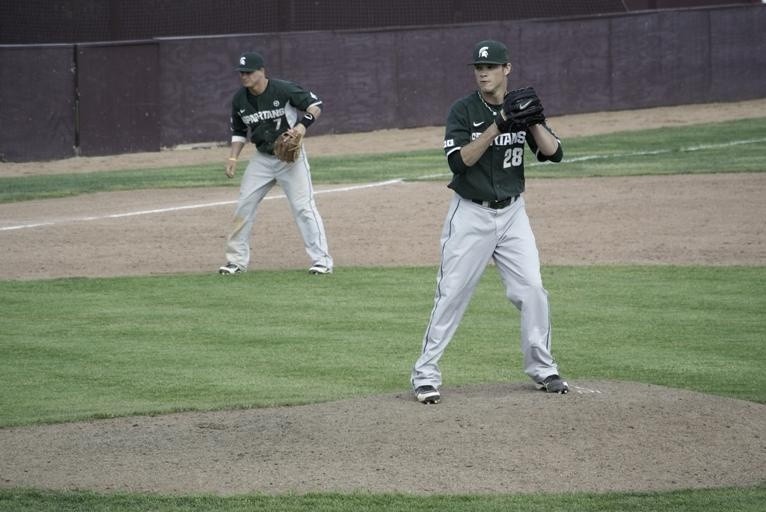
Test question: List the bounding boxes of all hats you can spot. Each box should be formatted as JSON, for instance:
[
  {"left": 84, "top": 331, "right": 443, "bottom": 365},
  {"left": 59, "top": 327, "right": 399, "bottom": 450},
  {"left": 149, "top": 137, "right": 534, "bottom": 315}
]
[
  {"left": 470, "top": 40, "right": 510, "bottom": 65},
  {"left": 233, "top": 52, "right": 265, "bottom": 73}
]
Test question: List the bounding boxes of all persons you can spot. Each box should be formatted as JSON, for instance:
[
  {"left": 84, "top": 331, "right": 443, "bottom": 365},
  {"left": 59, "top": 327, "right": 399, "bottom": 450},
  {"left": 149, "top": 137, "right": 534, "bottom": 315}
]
[
  {"left": 218, "top": 52, "right": 334, "bottom": 275},
  {"left": 410, "top": 40, "right": 568, "bottom": 403}
]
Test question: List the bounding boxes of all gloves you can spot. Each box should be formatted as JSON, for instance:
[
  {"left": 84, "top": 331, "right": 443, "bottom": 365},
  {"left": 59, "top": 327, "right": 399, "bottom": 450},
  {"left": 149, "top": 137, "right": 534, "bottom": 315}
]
[{"left": 494, "top": 87, "right": 545, "bottom": 136}]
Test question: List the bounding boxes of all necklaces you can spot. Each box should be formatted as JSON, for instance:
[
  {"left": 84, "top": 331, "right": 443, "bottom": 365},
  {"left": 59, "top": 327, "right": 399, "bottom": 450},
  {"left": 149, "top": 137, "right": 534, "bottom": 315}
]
[{"left": 478, "top": 90, "right": 507, "bottom": 116}]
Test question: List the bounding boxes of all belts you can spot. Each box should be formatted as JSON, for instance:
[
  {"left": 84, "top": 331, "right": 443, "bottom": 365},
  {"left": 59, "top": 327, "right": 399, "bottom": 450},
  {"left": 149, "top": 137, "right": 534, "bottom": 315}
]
[{"left": 471, "top": 194, "right": 521, "bottom": 209}]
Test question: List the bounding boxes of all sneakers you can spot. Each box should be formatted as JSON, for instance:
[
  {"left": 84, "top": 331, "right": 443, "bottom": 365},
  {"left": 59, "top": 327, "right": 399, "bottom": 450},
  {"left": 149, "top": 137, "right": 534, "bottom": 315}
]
[
  {"left": 219, "top": 264, "right": 247, "bottom": 275},
  {"left": 535, "top": 375, "right": 570, "bottom": 394},
  {"left": 309, "top": 264, "right": 332, "bottom": 275},
  {"left": 415, "top": 386, "right": 440, "bottom": 404}
]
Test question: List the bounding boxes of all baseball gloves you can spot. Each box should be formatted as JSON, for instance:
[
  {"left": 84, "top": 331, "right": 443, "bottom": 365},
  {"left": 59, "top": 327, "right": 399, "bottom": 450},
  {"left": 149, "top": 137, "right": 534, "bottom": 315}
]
[
  {"left": 494, "top": 86, "right": 545, "bottom": 133},
  {"left": 273, "top": 128, "right": 303, "bottom": 164}
]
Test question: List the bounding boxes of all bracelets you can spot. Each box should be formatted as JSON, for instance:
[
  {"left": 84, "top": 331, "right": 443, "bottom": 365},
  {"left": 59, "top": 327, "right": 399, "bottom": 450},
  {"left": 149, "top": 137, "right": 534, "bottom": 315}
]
[
  {"left": 299, "top": 112, "right": 315, "bottom": 129},
  {"left": 228, "top": 158, "right": 238, "bottom": 161}
]
